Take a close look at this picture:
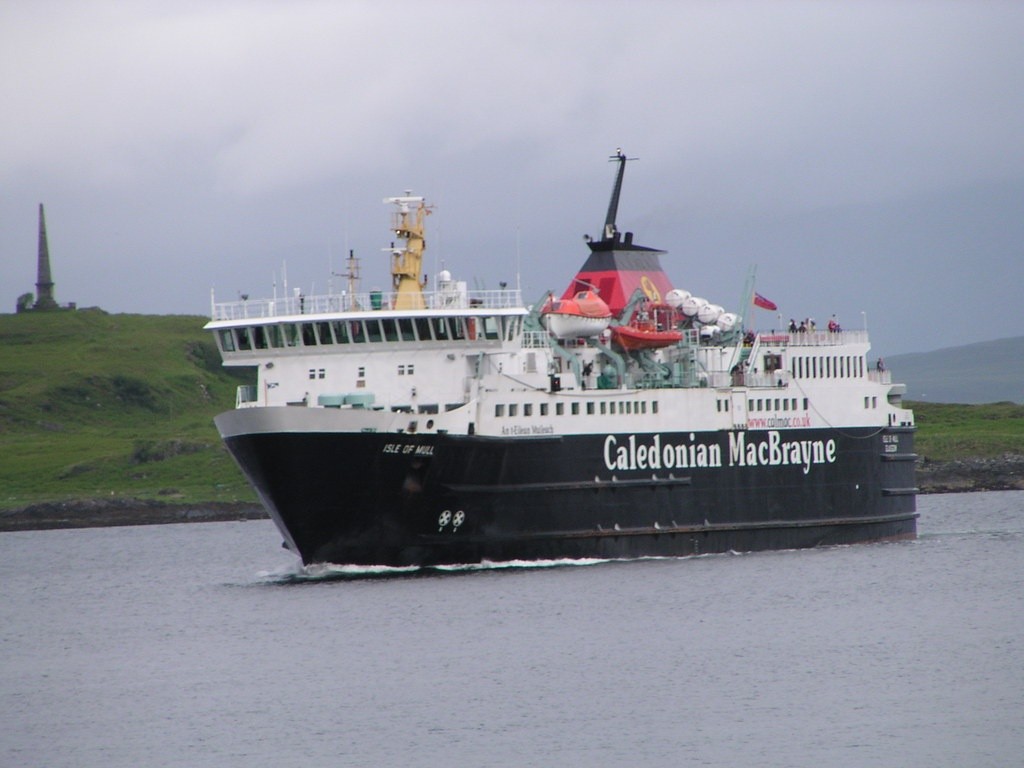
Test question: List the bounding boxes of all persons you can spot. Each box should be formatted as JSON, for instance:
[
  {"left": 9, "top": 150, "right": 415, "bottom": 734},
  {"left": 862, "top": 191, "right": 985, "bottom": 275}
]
[
  {"left": 730, "top": 358, "right": 792, "bottom": 388},
  {"left": 876, "top": 357, "right": 886, "bottom": 374},
  {"left": 740, "top": 317, "right": 846, "bottom": 347},
  {"left": 582, "top": 361, "right": 593, "bottom": 389}
]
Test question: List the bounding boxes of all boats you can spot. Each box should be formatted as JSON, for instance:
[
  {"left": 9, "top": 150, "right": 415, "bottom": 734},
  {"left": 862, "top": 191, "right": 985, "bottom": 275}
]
[
  {"left": 607, "top": 300, "right": 684, "bottom": 351},
  {"left": 203, "top": 147, "right": 920, "bottom": 574},
  {"left": 537, "top": 276, "right": 613, "bottom": 339}
]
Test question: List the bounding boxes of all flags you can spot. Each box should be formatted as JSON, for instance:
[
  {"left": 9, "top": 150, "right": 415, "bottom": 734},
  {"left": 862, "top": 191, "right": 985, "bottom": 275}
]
[{"left": 752, "top": 292, "right": 779, "bottom": 311}]
